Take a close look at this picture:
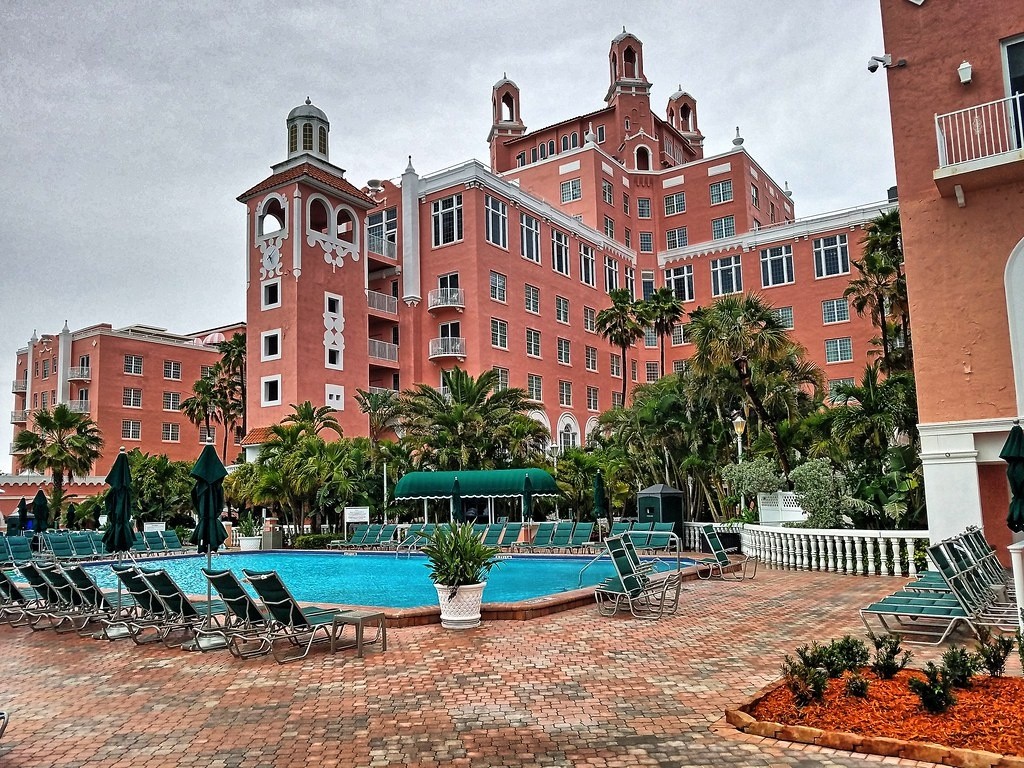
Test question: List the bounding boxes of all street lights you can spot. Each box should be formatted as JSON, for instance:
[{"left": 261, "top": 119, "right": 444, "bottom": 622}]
[
  {"left": 550, "top": 439, "right": 559, "bottom": 522},
  {"left": 380, "top": 446, "right": 389, "bottom": 525},
  {"left": 732, "top": 416, "right": 747, "bottom": 521}
]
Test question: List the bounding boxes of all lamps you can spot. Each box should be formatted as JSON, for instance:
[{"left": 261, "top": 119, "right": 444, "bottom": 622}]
[{"left": 956, "top": 60, "right": 972, "bottom": 84}]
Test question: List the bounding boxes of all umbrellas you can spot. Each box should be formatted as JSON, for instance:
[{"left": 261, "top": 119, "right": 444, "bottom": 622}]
[
  {"left": 94, "top": 503, "right": 102, "bottom": 530},
  {"left": 100, "top": 446, "right": 138, "bottom": 628},
  {"left": 522, "top": 472, "right": 536, "bottom": 544},
  {"left": 593, "top": 469, "right": 605, "bottom": 543},
  {"left": 998, "top": 418, "right": 1024, "bottom": 536},
  {"left": 66, "top": 501, "right": 76, "bottom": 529},
  {"left": 17, "top": 495, "right": 28, "bottom": 536},
  {"left": 189, "top": 436, "right": 229, "bottom": 626},
  {"left": 450, "top": 477, "right": 464, "bottom": 531},
  {"left": 29, "top": 487, "right": 50, "bottom": 552}
]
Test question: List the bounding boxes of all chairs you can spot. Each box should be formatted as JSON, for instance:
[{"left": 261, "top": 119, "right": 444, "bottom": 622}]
[
  {"left": 859, "top": 525, "right": 1023, "bottom": 647},
  {"left": 695, "top": 524, "right": 758, "bottom": 582},
  {"left": 0, "top": 516, "right": 683, "bottom": 664}
]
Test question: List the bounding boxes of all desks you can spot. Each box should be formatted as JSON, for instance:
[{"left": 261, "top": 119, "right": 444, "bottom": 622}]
[
  {"left": 331, "top": 609, "right": 387, "bottom": 658},
  {"left": 511, "top": 541, "right": 529, "bottom": 554},
  {"left": 580, "top": 541, "right": 602, "bottom": 555},
  {"left": 379, "top": 540, "right": 398, "bottom": 551},
  {"left": 329, "top": 540, "right": 348, "bottom": 550}
]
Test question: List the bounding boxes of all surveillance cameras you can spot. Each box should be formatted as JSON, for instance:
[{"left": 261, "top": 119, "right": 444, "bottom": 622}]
[{"left": 868, "top": 60, "right": 879, "bottom": 73}]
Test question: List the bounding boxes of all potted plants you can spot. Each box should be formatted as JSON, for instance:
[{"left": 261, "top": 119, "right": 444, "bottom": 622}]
[
  {"left": 237, "top": 511, "right": 263, "bottom": 551},
  {"left": 415, "top": 516, "right": 509, "bottom": 629}
]
